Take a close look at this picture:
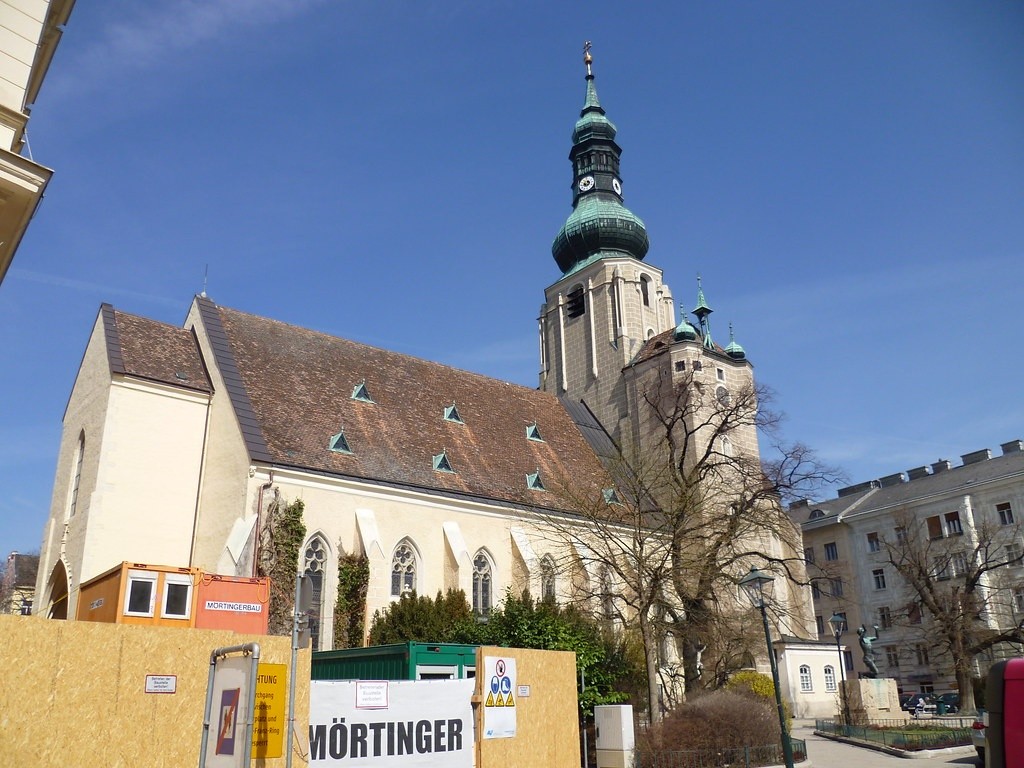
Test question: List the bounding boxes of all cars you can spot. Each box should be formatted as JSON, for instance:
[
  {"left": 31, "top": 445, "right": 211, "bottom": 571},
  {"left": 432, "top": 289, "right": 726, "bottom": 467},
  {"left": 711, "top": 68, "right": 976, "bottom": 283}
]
[
  {"left": 929, "top": 692, "right": 960, "bottom": 713},
  {"left": 899, "top": 692, "right": 915, "bottom": 707},
  {"left": 972, "top": 708, "right": 985, "bottom": 760},
  {"left": 902, "top": 694, "right": 944, "bottom": 716}
]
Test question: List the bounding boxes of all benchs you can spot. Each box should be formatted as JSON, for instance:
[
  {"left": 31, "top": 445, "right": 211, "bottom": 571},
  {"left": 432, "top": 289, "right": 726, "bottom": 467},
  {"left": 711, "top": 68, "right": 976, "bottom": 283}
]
[{"left": 914, "top": 705, "right": 951, "bottom": 720}]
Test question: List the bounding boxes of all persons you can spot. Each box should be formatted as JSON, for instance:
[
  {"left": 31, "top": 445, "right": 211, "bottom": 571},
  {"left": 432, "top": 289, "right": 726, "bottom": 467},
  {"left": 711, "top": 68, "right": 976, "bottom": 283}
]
[{"left": 857, "top": 623, "right": 880, "bottom": 679}]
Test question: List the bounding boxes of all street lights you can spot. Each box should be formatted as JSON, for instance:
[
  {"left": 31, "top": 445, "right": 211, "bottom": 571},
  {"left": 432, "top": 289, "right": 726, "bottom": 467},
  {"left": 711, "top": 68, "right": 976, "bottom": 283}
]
[
  {"left": 737, "top": 566, "right": 795, "bottom": 768},
  {"left": 827, "top": 611, "right": 853, "bottom": 735}
]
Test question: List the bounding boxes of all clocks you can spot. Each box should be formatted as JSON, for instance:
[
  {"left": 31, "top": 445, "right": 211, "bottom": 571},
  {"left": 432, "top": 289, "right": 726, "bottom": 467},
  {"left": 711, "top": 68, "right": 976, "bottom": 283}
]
[
  {"left": 580, "top": 175, "right": 594, "bottom": 192},
  {"left": 612, "top": 178, "right": 622, "bottom": 195}
]
